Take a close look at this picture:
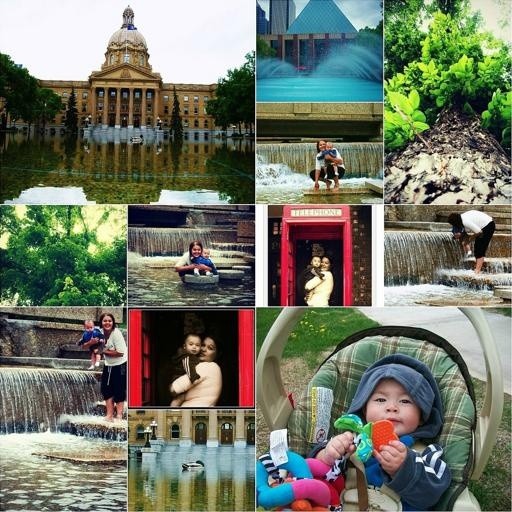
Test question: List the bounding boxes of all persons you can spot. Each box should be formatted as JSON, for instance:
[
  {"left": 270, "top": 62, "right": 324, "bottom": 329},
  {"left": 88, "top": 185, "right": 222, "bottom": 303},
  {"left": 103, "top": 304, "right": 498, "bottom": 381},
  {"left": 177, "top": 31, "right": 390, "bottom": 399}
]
[
  {"left": 166, "top": 332, "right": 224, "bottom": 407},
  {"left": 301, "top": 254, "right": 326, "bottom": 302},
  {"left": 316, "top": 141, "right": 340, "bottom": 179},
  {"left": 256, "top": 412, "right": 401, "bottom": 512},
  {"left": 95, "top": 311, "right": 129, "bottom": 421},
  {"left": 447, "top": 209, "right": 496, "bottom": 273},
  {"left": 450, "top": 225, "right": 473, "bottom": 257},
  {"left": 190, "top": 248, "right": 219, "bottom": 278},
  {"left": 303, "top": 255, "right": 335, "bottom": 307},
  {"left": 163, "top": 331, "right": 202, "bottom": 406},
  {"left": 174, "top": 240, "right": 204, "bottom": 282},
  {"left": 304, "top": 350, "right": 452, "bottom": 512},
  {"left": 309, "top": 141, "right": 345, "bottom": 192},
  {"left": 76, "top": 319, "right": 107, "bottom": 371}
]
